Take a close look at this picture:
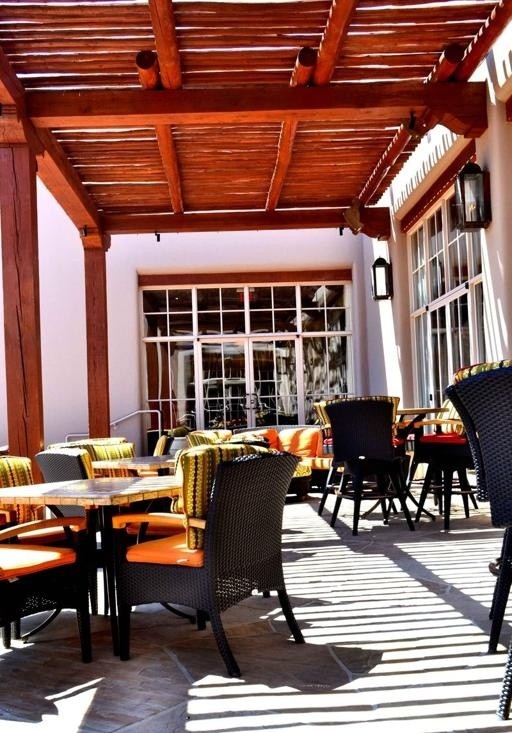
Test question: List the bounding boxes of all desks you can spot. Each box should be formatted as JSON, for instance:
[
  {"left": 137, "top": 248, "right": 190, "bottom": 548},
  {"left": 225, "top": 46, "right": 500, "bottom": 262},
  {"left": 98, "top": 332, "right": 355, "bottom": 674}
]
[{"left": 1, "top": 474, "right": 198, "bottom": 657}]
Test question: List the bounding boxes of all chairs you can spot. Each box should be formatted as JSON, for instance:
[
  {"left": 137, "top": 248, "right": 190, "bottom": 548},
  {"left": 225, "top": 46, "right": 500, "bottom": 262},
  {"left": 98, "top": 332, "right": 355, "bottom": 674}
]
[
  {"left": 117, "top": 440, "right": 308, "bottom": 681},
  {"left": 446, "top": 357, "right": 512, "bottom": 719},
  {"left": 1, "top": 522, "right": 96, "bottom": 665},
  {"left": 1, "top": 389, "right": 479, "bottom": 537}
]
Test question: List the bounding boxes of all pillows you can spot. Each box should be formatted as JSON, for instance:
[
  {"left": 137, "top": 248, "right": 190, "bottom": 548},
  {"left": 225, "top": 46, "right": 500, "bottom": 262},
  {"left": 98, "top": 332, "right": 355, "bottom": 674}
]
[{"left": 170, "top": 442, "right": 282, "bottom": 550}]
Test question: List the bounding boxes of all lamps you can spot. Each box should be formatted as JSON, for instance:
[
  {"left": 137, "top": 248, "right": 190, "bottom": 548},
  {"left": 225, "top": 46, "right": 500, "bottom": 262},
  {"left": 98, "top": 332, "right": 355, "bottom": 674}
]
[
  {"left": 369, "top": 251, "right": 396, "bottom": 304},
  {"left": 451, "top": 158, "right": 492, "bottom": 237}
]
